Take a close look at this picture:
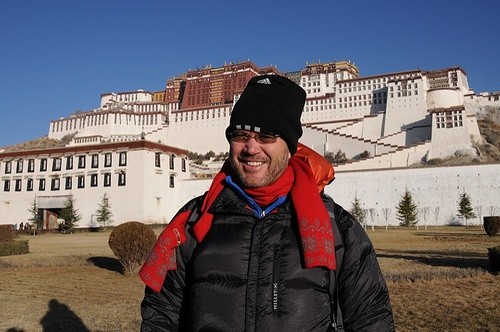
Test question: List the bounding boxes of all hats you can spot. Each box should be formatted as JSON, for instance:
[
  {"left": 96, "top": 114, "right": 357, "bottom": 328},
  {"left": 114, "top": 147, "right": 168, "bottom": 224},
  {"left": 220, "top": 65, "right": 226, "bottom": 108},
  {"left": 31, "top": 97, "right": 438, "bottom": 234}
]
[{"left": 225, "top": 75, "right": 307, "bottom": 158}]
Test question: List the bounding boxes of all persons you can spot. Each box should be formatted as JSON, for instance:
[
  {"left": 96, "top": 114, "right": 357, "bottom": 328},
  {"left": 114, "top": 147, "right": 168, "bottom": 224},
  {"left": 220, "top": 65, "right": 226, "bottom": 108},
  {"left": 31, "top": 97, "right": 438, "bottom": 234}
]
[
  {"left": 139, "top": 75, "right": 397, "bottom": 331},
  {"left": 58, "top": 221, "right": 64, "bottom": 232},
  {"left": 19, "top": 222, "right": 31, "bottom": 230}
]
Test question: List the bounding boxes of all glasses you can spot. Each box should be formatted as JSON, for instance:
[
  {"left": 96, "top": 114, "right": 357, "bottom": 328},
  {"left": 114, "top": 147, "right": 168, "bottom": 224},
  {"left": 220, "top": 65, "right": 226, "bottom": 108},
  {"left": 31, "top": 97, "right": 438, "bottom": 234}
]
[{"left": 227, "top": 130, "right": 283, "bottom": 144}]
